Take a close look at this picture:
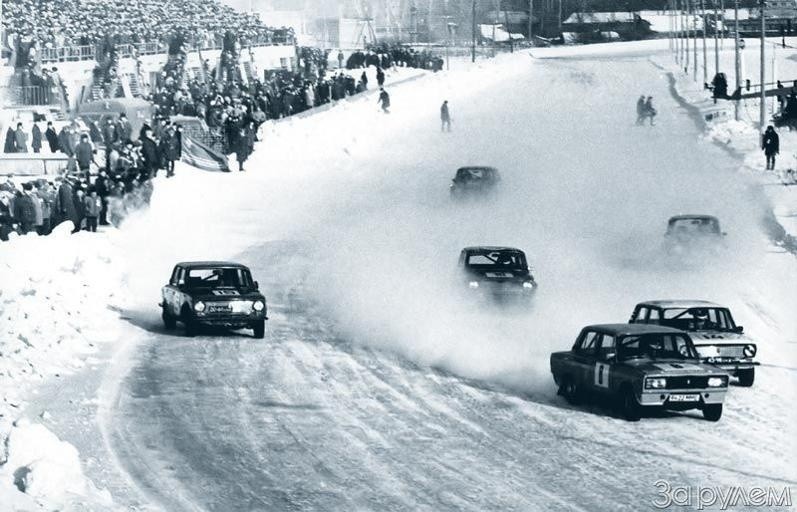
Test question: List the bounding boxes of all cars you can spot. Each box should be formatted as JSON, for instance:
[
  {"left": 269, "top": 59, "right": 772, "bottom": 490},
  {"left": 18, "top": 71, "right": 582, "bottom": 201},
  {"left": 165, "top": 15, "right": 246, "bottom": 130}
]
[
  {"left": 545, "top": 320, "right": 733, "bottom": 423},
  {"left": 661, "top": 213, "right": 728, "bottom": 258},
  {"left": 156, "top": 259, "right": 267, "bottom": 342},
  {"left": 447, "top": 167, "right": 501, "bottom": 201},
  {"left": 170, "top": 115, "right": 225, "bottom": 155},
  {"left": 450, "top": 246, "right": 536, "bottom": 306}
]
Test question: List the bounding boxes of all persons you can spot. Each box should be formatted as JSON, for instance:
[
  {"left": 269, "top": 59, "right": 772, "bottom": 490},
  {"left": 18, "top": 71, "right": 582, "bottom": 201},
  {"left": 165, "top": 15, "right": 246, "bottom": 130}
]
[
  {"left": 636, "top": 95, "right": 656, "bottom": 126},
  {"left": 761, "top": 125, "right": 779, "bottom": 170}
]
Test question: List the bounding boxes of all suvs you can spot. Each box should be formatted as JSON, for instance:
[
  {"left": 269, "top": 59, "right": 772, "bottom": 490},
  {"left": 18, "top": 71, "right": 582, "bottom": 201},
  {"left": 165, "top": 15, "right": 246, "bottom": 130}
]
[{"left": 620, "top": 296, "right": 761, "bottom": 389}]
